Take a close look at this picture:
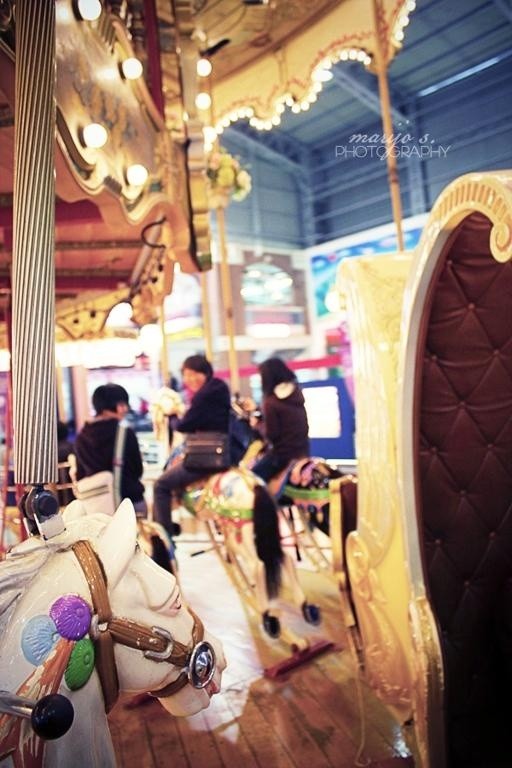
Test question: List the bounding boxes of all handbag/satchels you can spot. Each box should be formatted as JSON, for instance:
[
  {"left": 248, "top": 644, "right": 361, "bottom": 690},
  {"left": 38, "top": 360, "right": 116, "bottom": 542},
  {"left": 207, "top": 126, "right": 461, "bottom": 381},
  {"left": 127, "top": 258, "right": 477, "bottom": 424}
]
[{"left": 183, "top": 432, "right": 230, "bottom": 470}]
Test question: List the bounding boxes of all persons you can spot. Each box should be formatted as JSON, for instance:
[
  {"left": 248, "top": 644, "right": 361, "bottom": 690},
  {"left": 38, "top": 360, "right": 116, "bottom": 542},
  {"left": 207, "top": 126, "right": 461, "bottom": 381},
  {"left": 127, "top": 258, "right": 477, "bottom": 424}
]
[
  {"left": 67, "top": 455, "right": 181, "bottom": 595},
  {"left": 57, "top": 419, "right": 74, "bottom": 462},
  {"left": 75, "top": 381, "right": 149, "bottom": 520},
  {"left": 250, "top": 356, "right": 311, "bottom": 485},
  {"left": 152, "top": 355, "right": 233, "bottom": 549}
]
